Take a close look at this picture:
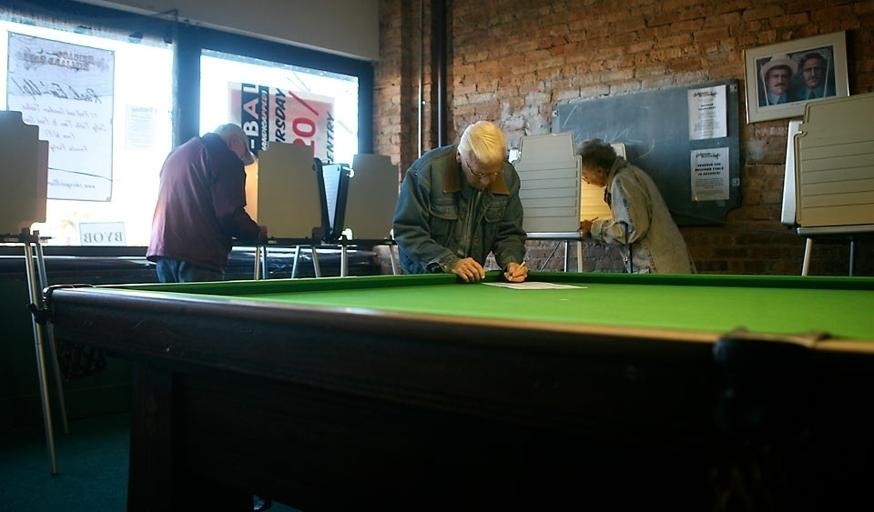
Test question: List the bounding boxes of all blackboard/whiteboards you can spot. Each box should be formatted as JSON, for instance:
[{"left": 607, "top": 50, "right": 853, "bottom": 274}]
[{"left": 552, "top": 79, "right": 743, "bottom": 228}]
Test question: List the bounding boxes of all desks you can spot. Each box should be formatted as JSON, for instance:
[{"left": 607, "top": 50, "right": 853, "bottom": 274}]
[{"left": 28, "top": 264, "right": 873, "bottom": 510}]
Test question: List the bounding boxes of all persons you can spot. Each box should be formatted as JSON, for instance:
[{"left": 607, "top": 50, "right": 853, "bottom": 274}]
[
  {"left": 793, "top": 51, "right": 828, "bottom": 101},
  {"left": 759, "top": 55, "right": 800, "bottom": 108},
  {"left": 145, "top": 123, "right": 268, "bottom": 283},
  {"left": 575, "top": 139, "right": 698, "bottom": 274},
  {"left": 392, "top": 119, "right": 528, "bottom": 283}
]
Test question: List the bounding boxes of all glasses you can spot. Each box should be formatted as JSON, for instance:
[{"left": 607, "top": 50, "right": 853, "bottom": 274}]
[
  {"left": 581, "top": 176, "right": 594, "bottom": 183},
  {"left": 465, "top": 160, "right": 506, "bottom": 177}
]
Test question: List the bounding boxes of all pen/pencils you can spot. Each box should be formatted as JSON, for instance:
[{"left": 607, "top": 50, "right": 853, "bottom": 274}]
[
  {"left": 576, "top": 216, "right": 599, "bottom": 232},
  {"left": 509, "top": 261, "right": 526, "bottom": 281}
]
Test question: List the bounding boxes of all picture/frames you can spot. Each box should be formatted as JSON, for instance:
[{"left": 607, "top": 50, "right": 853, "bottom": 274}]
[{"left": 742, "top": 27, "right": 854, "bottom": 126}]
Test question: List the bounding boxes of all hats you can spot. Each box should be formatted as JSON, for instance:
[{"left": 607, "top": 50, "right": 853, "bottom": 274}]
[
  {"left": 214, "top": 123, "right": 254, "bottom": 166},
  {"left": 761, "top": 54, "right": 799, "bottom": 83}
]
